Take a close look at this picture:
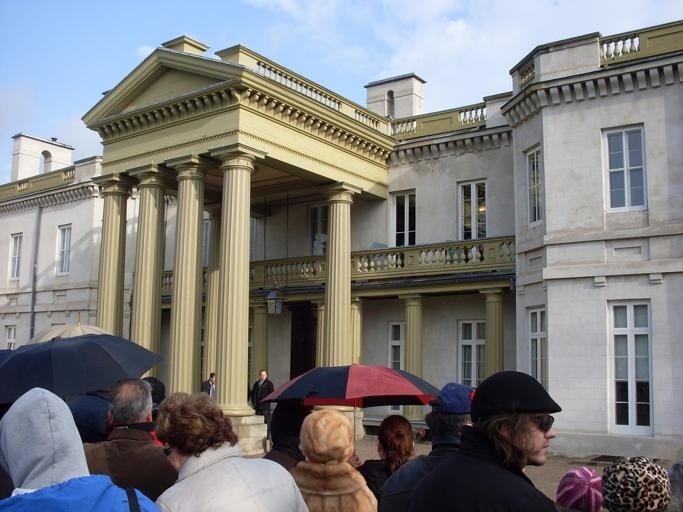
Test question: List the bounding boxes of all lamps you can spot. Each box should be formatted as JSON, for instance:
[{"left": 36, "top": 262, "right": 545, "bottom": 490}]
[{"left": 260, "top": 281, "right": 288, "bottom": 320}]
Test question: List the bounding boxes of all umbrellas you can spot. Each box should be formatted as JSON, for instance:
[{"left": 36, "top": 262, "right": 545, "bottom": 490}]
[
  {"left": 256, "top": 363, "right": 441, "bottom": 459},
  {"left": 0, "top": 333, "right": 164, "bottom": 407},
  {"left": 25, "top": 312, "right": 114, "bottom": 346}
]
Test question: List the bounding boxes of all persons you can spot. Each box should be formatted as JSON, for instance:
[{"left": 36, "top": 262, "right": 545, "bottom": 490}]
[
  {"left": 403, "top": 369, "right": 557, "bottom": 510},
  {"left": 201, "top": 373, "right": 215, "bottom": 398},
  {"left": 553, "top": 465, "right": 603, "bottom": 511},
  {"left": 1, "top": 376, "right": 414, "bottom": 512},
  {"left": 251, "top": 370, "right": 274, "bottom": 437},
  {"left": 600, "top": 453, "right": 672, "bottom": 511},
  {"left": 378, "top": 380, "right": 475, "bottom": 510}
]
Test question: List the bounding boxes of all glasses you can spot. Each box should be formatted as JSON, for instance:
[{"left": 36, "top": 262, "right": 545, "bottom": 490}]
[{"left": 532, "top": 416, "right": 554, "bottom": 431}]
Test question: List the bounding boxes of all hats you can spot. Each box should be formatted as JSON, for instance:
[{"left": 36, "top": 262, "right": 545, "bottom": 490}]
[
  {"left": 299, "top": 409, "right": 355, "bottom": 463},
  {"left": 557, "top": 457, "right": 671, "bottom": 512},
  {"left": 429, "top": 371, "right": 561, "bottom": 421}
]
